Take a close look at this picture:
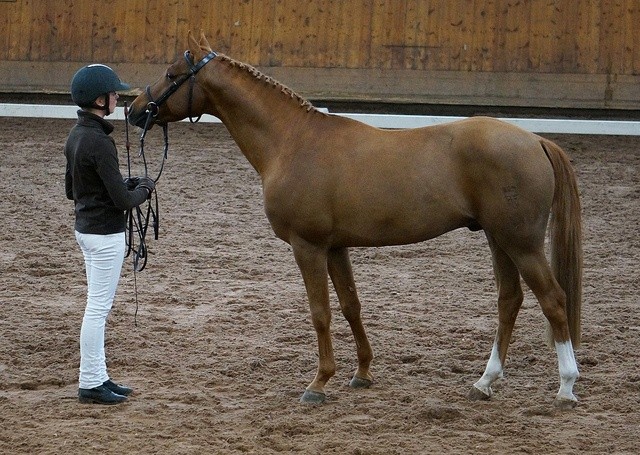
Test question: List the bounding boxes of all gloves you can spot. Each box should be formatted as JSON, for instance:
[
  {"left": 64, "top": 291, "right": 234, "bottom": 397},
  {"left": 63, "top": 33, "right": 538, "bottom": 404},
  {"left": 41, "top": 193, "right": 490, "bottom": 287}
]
[{"left": 124, "top": 177, "right": 155, "bottom": 193}]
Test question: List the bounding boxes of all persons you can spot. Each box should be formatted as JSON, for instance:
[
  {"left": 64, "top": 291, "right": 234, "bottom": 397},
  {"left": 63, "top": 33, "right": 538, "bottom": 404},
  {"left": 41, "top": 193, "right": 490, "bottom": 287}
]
[{"left": 62, "top": 64, "right": 154, "bottom": 405}]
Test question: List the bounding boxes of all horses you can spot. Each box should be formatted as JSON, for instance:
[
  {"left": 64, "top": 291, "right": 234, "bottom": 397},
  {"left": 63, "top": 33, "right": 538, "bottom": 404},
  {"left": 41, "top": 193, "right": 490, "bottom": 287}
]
[{"left": 126, "top": 27, "right": 586, "bottom": 413}]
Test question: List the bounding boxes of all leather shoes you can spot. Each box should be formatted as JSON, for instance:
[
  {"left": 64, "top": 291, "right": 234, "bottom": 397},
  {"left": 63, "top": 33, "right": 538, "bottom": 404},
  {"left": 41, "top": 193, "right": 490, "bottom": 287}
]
[
  {"left": 103, "top": 379, "right": 133, "bottom": 395},
  {"left": 79, "top": 385, "right": 127, "bottom": 404}
]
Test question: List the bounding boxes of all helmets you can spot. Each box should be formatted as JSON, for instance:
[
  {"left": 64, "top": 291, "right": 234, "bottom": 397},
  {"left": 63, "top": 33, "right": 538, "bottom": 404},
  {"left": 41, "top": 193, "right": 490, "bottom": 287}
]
[{"left": 71, "top": 64, "right": 129, "bottom": 104}]
